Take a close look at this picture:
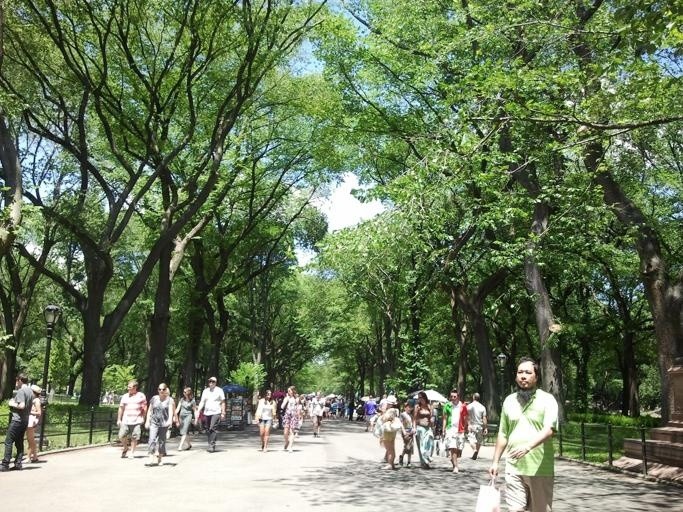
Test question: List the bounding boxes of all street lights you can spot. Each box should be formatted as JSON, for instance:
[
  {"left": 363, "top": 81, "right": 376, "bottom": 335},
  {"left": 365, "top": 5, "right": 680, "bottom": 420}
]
[
  {"left": 422, "top": 370, "right": 429, "bottom": 391},
  {"left": 36, "top": 301, "right": 62, "bottom": 452},
  {"left": 495, "top": 351, "right": 507, "bottom": 408},
  {"left": 244, "top": 375, "right": 249, "bottom": 395},
  {"left": 194, "top": 358, "right": 201, "bottom": 403}
]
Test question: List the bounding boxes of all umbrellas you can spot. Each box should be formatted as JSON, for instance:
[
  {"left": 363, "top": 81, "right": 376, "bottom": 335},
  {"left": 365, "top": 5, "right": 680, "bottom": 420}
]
[{"left": 223, "top": 382, "right": 250, "bottom": 394}]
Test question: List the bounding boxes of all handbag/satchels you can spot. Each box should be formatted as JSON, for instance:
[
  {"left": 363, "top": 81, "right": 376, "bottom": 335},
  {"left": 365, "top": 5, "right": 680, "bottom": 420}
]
[{"left": 474, "top": 472, "right": 502, "bottom": 512}]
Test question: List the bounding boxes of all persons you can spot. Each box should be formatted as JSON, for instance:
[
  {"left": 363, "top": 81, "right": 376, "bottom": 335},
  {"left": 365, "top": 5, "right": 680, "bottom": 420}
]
[
  {"left": 172, "top": 387, "right": 198, "bottom": 451},
  {"left": 154, "top": 388, "right": 179, "bottom": 458},
  {"left": 196, "top": 376, "right": 226, "bottom": 452},
  {"left": 489, "top": 356, "right": 560, "bottom": 512},
  {"left": 25, "top": 384, "right": 42, "bottom": 463},
  {"left": 0, "top": 373, "right": 32, "bottom": 471},
  {"left": 144, "top": 383, "right": 173, "bottom": 466},
  {"left": 255, "top": 386, "right": 488, "bottom": 474},
  {"left": 117, "top": 380, "right": 148, "bottom": 458}
]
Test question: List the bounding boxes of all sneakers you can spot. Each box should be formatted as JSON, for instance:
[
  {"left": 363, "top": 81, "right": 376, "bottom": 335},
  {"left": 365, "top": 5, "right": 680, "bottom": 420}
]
[
  {"left": 261, "top": 444, "right": 295, "bottom": 453},
  {"left": 379, "top": 450, "right": 480, "bottom": 473},
  {"left": 121, "top": 444, "right": 216, "bottom": 467},
  {"left": 1, "top": 457, "right": 41, "bottom": 472}
]
